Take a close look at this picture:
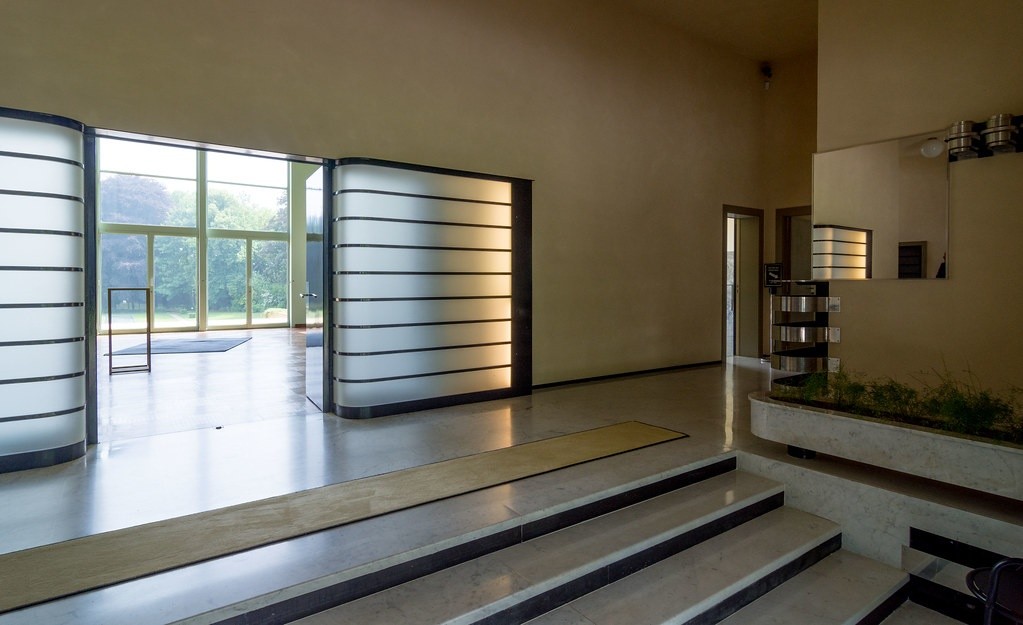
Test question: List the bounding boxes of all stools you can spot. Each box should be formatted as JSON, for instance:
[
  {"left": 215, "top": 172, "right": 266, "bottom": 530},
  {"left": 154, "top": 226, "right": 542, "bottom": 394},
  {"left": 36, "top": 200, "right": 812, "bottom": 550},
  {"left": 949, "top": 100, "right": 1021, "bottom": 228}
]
[{"left": 968, "top": 558, "right": 1023, "bottom": 625}]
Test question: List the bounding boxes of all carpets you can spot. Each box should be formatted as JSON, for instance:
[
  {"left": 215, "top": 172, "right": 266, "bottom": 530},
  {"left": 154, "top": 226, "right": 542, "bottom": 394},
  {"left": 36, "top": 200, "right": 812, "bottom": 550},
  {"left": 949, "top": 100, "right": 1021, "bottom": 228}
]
[{"left": 102, "top": 336, "right": 253, "bottom": 355}]
